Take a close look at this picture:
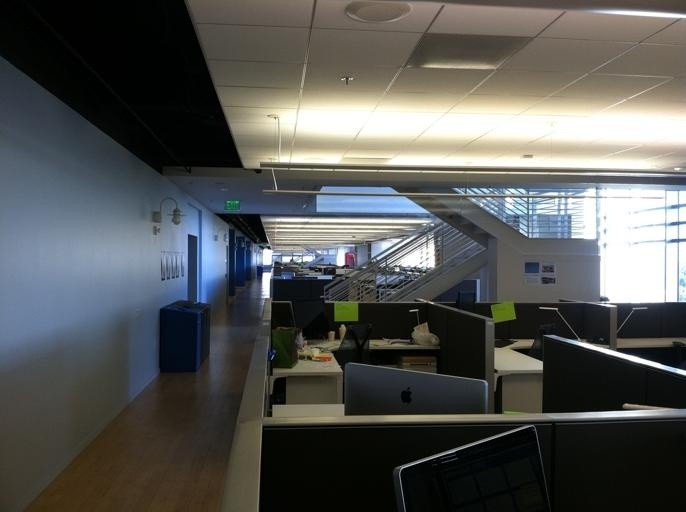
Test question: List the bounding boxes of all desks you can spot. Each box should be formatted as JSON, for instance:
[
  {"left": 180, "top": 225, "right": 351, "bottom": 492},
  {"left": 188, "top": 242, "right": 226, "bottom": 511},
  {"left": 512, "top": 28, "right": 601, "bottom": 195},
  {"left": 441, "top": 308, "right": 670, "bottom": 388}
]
[{"left": 216, "top": 260, "right": 686, "bottom": 512}]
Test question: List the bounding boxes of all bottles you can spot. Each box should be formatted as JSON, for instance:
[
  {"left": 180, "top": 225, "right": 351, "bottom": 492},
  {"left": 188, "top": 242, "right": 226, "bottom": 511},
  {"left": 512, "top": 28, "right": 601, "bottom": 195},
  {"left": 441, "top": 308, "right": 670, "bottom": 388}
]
[
  {"left": 298, "top": 350, "right": 305, "bottom": 359},
  {"left": 339, "top": 324, "right": 346, "bottom": 341}
]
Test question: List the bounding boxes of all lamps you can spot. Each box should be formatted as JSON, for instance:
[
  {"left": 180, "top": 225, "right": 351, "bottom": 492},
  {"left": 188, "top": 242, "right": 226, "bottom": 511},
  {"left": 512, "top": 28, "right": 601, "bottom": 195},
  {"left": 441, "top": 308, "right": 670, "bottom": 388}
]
[{"left": 152, "top": 196, "right": 187, "bottom": 226}]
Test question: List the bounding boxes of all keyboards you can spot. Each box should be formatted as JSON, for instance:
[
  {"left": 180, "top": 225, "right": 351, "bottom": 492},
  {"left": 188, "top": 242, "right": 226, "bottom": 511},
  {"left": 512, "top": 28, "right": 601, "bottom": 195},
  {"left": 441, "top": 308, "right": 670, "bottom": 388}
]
[
  {"left": 494, "top": 338, "right": 514, "bottom": 348},
  {"left": 314, "top": 340, "right": 341, "bottom": 349}
]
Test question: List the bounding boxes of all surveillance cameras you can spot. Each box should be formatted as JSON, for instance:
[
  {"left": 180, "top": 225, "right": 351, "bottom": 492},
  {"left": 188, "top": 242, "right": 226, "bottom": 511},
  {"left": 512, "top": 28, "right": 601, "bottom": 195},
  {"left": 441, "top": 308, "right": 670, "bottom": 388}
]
[{"left": 254, "top": 169, "right": 262, "bottom": 174}]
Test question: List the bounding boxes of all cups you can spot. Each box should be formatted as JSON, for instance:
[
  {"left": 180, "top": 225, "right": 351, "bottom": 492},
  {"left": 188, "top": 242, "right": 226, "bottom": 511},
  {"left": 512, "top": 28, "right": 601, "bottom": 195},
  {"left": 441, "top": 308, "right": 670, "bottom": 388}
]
[
  {"left": 306, "top": 352, "right": 312, "bottom": 359},
  {"left": 328, "top": 332, "right": 335, "bottom": 341},
  {"left": 311, "top": 347, "right": 320, "bottom": 361}
]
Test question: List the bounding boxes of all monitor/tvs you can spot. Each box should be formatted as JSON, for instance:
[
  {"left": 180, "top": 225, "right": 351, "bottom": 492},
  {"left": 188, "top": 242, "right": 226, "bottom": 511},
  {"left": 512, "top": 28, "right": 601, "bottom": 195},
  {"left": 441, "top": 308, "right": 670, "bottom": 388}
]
[
  {"left": 292, "top": 297, "right": 330, "bottom": 339},
  {"left": 393, "top": 424, "right": 551, "bottom": 512},
  {"left": 459, "top": 291, "right": 475, "bottom": 314},
  {"left": 344, "top": 362, "right": 488, "bottom": 416}
]
